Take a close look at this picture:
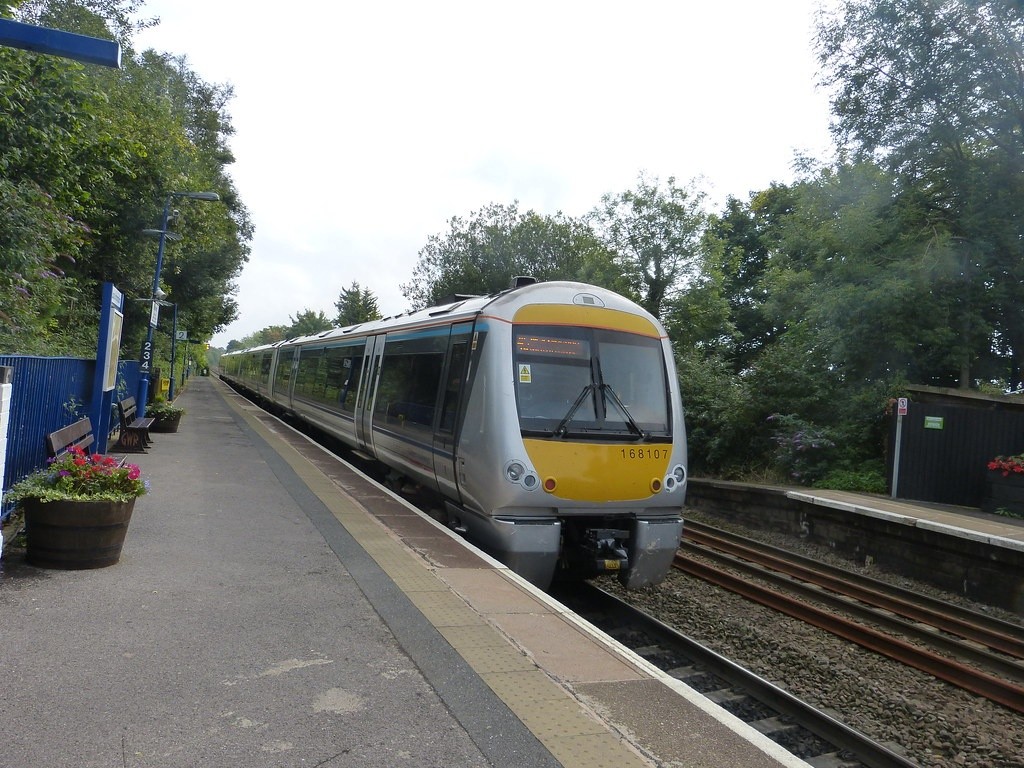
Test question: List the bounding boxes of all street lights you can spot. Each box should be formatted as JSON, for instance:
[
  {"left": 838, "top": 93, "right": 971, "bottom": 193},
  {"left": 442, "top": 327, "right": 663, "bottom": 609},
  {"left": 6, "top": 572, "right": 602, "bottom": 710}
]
[{"left": 135, "top": 192, "right": 219, "bottom": 417}]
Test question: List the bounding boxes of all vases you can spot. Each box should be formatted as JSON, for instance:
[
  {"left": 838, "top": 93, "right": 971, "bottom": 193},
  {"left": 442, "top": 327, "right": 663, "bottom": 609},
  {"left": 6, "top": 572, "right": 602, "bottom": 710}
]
[
  {"left": 152, "top": 412, "right": 181, "bottom": 433},
  {"left": 982, "top": 469, "right": 1024, "bottom": 517},
  {"left": 21, "top": 494, "right": 137, "bottom": 568}
]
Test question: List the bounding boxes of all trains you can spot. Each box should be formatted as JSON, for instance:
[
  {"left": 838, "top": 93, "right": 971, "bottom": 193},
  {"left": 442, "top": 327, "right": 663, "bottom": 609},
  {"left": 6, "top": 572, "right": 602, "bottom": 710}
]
[{"left": 218, "top": 276, "right": 688, "bottom": 592}]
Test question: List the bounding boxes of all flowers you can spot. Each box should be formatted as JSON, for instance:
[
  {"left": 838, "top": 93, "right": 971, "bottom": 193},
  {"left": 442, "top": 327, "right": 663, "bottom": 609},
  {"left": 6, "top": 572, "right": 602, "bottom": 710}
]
[
  {"left": 145, "top": 394, "right": 187, "bottom": 422},
  {"left": 985, "top": 453, "right": 1024, "bottom": 476},
  {"left": 4, "top": 446, "right": 151, "bottom": 503}
]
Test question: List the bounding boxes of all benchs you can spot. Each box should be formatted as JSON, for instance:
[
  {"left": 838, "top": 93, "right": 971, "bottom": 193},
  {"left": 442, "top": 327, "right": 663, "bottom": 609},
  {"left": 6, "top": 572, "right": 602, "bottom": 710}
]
[
  {"left": 120, "top": 396, "right": 155, "bottom": 454},
  {"left": 49, "top": 417, "right": 127, "bottom": 472}
]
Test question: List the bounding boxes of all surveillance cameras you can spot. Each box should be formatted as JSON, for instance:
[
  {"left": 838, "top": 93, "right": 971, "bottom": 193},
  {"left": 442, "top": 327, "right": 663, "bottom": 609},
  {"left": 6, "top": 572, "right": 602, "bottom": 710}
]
[
  {"left": 172, "top": 210, "right": 179, "bottom": 217},
  {"left": 157, "top": 290, "right": 166, "bottom": 300}
]
